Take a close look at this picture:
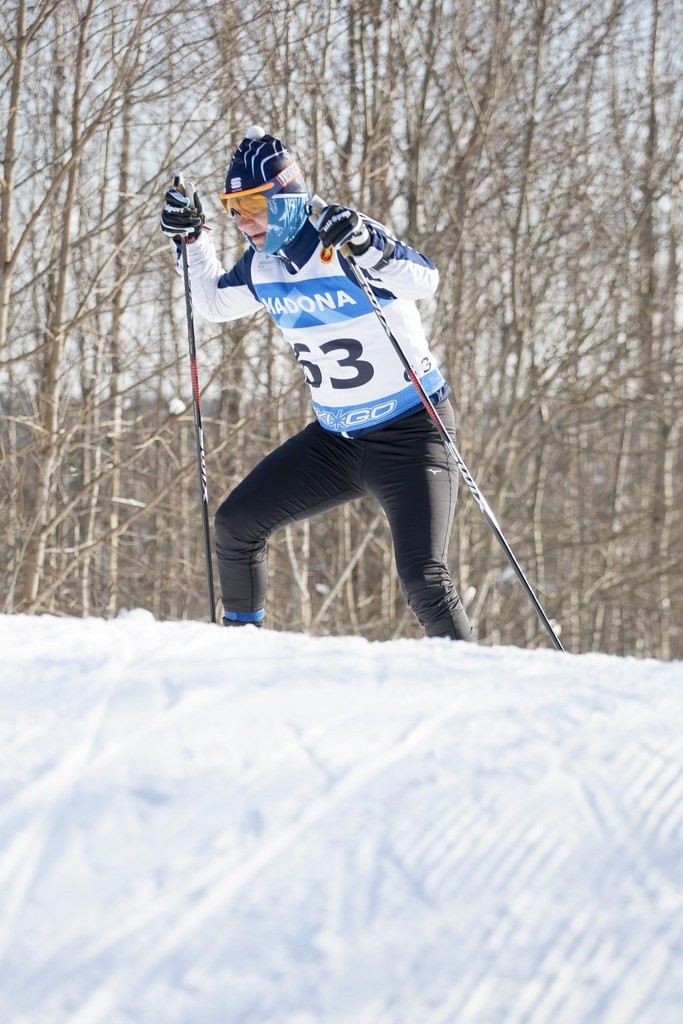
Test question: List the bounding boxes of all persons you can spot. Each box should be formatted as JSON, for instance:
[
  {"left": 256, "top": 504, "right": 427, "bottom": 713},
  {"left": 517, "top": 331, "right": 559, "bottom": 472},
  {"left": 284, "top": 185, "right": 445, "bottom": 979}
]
[{"left": 160, "top": 127, "right": 485, "bottom": 642}]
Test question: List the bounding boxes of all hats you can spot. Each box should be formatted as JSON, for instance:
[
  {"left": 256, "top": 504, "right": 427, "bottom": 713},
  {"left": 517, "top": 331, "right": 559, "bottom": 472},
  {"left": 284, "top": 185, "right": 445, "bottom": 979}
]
[{"left": 224, "top": 127, "right": 307, "bottom": 197}]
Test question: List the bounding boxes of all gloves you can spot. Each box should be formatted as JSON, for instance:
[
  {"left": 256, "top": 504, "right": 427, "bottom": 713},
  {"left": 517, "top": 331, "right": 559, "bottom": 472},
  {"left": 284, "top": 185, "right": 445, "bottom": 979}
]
[
  {"left": 160, "top": 182, "right": 205, "bottom": 243},
  {"left": 309, "top": 202, "right": 371, "bottom": 256}
]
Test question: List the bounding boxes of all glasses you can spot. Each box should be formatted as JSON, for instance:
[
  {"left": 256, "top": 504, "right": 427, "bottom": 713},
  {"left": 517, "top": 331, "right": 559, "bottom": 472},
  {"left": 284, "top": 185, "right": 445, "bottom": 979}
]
[{"left": 219, "top": 183, "right": 273, "bottom": 217}]
